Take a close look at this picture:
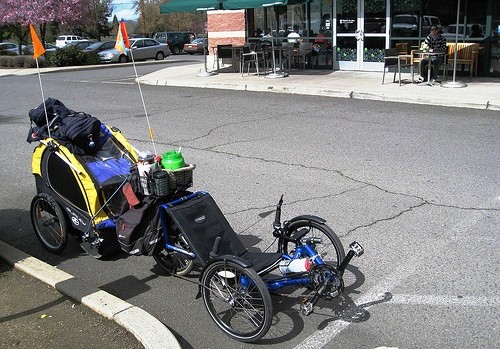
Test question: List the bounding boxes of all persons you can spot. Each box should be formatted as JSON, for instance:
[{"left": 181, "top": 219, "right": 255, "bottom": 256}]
[
  {"left": 190, "top": 33, "right": 195, "bottom": 44},
  {"left": 255, "top": 28, "right": 263, "bottom": 37},
  {"left": 264, "top": 29, "right": 274, "bottom": 37},
  {"left": 287, "top": 25, "right": 302, "bottom": 48},
  {"left": 469, "top": 24, "right": 485, "bottom": 38},
  {"left": 315, "top": 29, "right": 327, "bottom": 44},
  {"left": 416, "top": 24, "right": 446, "bottom": 86}
]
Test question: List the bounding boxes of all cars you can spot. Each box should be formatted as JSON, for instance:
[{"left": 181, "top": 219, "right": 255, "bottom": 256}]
[
  {"left": 0, "top": 43, "right": 19, "bottom": 56},
  {"left": 324, "top": 16, "right": 488, "bottom": 45},
  {"left": 22, "top": 43, "right": 56, "bottom": 55},
  {"left": 184, "top": 38, "right": 208, "bottom": 55},
  {"left": 82, "top": 41, "right": 117, "bottom": 53},
  {"left": 97, "top": 38, "right": 171, "bottom": 63},
  {"left": 63, "top": 41, "right": 95, "bottom": 50}
]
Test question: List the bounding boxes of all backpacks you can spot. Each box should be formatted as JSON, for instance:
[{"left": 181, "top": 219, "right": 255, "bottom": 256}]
[
  {"left": 116, "top": 197, "right": 161, "bottom": 256},
  {"left": 27, "top": 97, "right": 101, "bottom": 156}
]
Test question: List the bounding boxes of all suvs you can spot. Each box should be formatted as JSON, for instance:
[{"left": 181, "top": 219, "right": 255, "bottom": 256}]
[{"left": 56, "top": 35, "right": 88, "bottom": 49}]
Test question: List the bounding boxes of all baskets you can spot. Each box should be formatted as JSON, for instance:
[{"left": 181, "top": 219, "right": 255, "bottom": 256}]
[{"left": 137, "top": 163, "right": 196, "bottom": 196}]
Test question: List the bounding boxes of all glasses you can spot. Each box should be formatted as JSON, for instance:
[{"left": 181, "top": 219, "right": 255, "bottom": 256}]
[{"left": 431, "top": 28, "right": 437, "bottom": 31}]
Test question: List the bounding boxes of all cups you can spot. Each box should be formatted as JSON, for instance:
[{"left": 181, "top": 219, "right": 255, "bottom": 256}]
[
  {"left": 153, "top": 170, "right": 168, "bottom": 196},
  {"left": 278, "top": 258, "right": 310, "bottom": 274}
]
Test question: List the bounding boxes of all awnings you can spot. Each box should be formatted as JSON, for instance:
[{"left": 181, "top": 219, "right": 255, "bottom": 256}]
[{"left": 159, "top": 0, "right": 311, "bottom": 76}]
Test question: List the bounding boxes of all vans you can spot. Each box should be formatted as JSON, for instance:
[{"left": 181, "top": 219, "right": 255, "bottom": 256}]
[{"left": 155, "top": 31, "right": 196, "bottom": 55}]
